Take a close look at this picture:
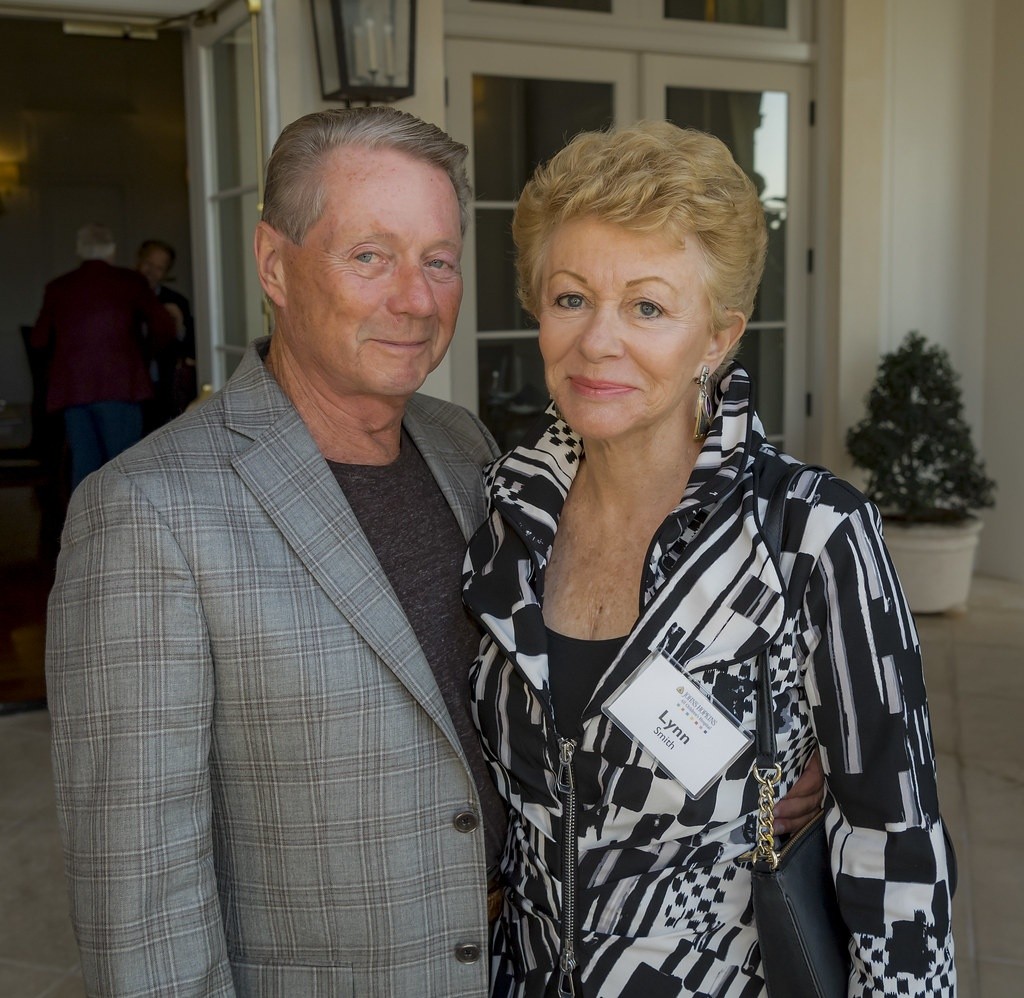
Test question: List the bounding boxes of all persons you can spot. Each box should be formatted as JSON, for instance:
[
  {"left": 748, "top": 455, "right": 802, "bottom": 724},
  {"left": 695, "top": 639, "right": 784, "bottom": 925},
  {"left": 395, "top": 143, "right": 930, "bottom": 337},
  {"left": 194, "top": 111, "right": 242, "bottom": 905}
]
[
  {"left": 459, "top": 120, "right": 957, "bottom": 998},
  {"left": 31, "top": 224, "right": 176, "bottom": 498},
  {"left": 46, "top": 107, "right": 832, "bottom": 998},
  {"left": 135, "top": 237, "right": 198, "bottom": 431}
]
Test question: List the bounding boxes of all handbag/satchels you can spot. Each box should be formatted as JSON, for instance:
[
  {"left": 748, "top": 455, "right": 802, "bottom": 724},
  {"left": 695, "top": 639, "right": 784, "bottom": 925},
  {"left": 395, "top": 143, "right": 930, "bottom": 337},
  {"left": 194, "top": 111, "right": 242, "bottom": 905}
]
[{"left": 748, "top": 466, "right": 852, "bottom": 997}]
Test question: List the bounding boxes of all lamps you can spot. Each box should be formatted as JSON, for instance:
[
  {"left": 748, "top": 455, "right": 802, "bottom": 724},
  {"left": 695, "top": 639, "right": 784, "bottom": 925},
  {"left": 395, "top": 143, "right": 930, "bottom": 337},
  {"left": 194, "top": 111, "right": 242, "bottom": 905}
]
[{"left": 310, "top": 0, "right": 416, "bottom": 109}]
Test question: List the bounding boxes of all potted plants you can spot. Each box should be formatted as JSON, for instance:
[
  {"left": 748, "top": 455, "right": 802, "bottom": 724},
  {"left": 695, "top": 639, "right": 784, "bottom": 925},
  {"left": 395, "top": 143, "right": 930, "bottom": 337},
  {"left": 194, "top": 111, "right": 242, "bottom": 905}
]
[{"left": 844, "top": 329, "right": 999, "bottom": 615}]
